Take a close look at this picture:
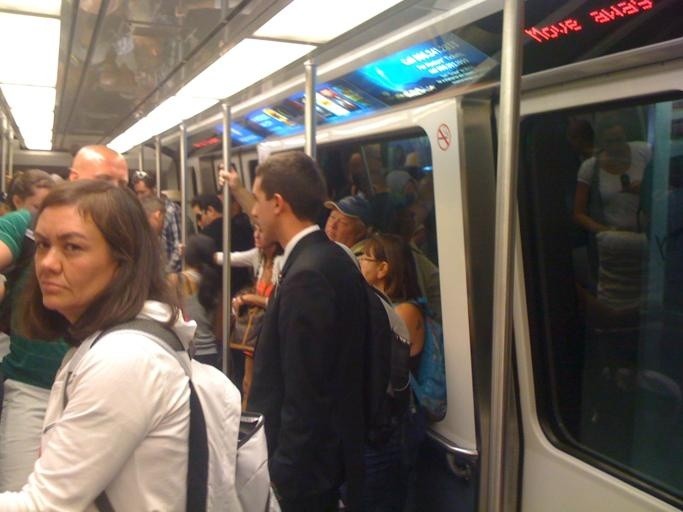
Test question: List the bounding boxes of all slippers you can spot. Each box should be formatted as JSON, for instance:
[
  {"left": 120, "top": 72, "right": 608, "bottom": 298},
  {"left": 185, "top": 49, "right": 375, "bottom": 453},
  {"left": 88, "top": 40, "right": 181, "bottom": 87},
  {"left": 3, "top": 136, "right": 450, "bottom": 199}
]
[{"left": 393, "top": 295, "right": 447, "bottom": 421}]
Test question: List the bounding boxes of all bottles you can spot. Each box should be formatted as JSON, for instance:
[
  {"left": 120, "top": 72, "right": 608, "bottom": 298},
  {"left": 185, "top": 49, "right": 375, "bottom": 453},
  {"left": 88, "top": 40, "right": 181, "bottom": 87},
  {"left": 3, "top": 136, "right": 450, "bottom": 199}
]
[{"left": 323, "top": 196, "right": 378, "bottom": 228}]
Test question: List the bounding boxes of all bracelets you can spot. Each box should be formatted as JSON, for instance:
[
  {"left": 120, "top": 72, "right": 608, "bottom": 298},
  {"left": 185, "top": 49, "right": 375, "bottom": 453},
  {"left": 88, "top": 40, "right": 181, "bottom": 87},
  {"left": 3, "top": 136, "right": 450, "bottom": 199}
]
[{"left": 240, "top": 293, "right": 248, "bottom": 304}]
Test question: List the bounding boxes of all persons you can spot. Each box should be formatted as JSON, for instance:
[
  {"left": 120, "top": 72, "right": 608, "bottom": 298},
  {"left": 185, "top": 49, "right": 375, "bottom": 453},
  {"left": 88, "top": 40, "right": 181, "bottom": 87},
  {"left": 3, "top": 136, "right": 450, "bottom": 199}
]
[
  {"left": 128, "top": 162, "right": 428, "bottom": 512},
  {"left": 2, "top": 179, "right": 244, "bottom": 512},
  {"left": 563, "top": 122, "right": 646, "bottom": 299},
  {"left": 2, "top": 146, "right": 128, "bottom": 494},
  {"left": 244, "top": 150, "right": 368, "bottom": 512},
  {"left": 2, "top": 168, "right": 64, "bottom": 357},
  {"left": 350, "top": 153, "right": 439, "bottom": 268}
]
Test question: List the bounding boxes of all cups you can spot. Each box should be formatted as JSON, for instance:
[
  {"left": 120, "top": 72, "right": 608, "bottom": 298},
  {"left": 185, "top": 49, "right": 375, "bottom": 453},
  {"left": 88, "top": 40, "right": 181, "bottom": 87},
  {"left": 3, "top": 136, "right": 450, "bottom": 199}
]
[
  {"left": 195, "top": 208, "right": 207, "bottom": 222},
  {"left": 357, "top": 255, "right": 379, "bottom": 265}
]
